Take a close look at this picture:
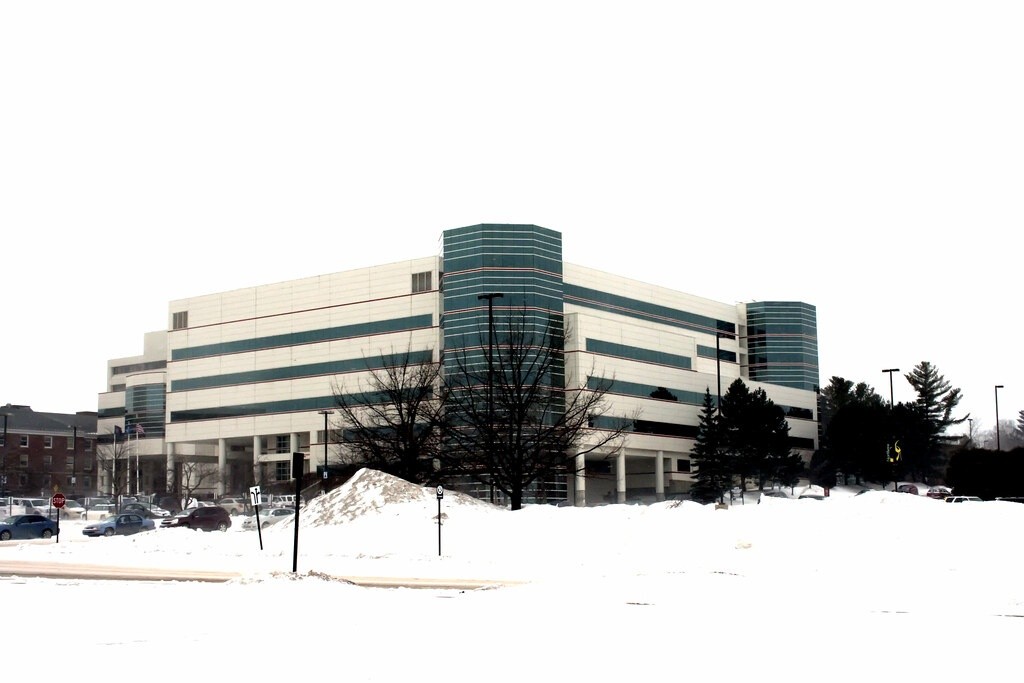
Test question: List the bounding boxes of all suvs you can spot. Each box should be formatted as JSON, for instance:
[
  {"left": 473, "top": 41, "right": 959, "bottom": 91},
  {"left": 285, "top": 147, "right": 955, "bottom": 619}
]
[
  {"left": 258, "top": 496, "right": 286, "bottom": 511},
  {"left": 216, "top": 497, "right": 251, "bottom": 516},
  {"left": 159, "top": 506, "right": 232, "bottom": 532}
]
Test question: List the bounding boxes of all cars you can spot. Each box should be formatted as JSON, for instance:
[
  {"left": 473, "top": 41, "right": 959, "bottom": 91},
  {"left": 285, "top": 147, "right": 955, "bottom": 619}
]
[
  {"left": 927, "top": 486, "right": 953, "bottom": 498},
  {"left": 854, "top": 487, "right": 874, "bottom": 496},
  {"left": 945, "top": 494, "right": 982, "bottom": 502},
  {"left": 82, "top": 513, "right": 156, "bottom": 537},
  {"left": 241, "top": 508, "right": 295, "bottom": 531},
  {"left": 0, "top": 495, "right": 216, "bottom": 520},
  {"left": 758, "top": 490, "right": 787, "bottom": 504},
  {"left": 0, "top": 514, "right": 60, "bottom": 541},
  {"left": 892, "top": 484, "right": 919, "bottom": 493},
  {"left": 994, "top": 496, "right": 1024, "bottom": 503},
  {"left": 799, "top": 493, "right": 824, "bottom": 500}
]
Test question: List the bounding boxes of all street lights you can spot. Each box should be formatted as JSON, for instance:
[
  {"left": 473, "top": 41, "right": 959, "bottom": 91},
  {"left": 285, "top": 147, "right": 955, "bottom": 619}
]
[
  {"left": 882, "top": 368, "right": 901, "bottom": 492},
  {"left": 994, "top": 384, "right": 1005, "bottom": 451},
  {"left": 967, "top": 418, "right": 974, "bottom": 450},
  {"left": 68, "top": 425, "right": 81, "bottom": 494},
  {"left": 317, "top": 410, "right": 335, "bottom": 493}
]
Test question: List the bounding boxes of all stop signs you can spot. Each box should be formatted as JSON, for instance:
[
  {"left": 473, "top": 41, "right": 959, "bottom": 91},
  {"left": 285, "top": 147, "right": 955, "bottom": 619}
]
[{"left": 52, "top": 493, "right": 66, "bottom": 508}]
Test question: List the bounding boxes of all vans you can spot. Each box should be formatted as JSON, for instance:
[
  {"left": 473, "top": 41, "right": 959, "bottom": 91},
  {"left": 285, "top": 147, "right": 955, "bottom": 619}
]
[{"left": 276, "top": 494, "right": 305, "bottom": 508}]
